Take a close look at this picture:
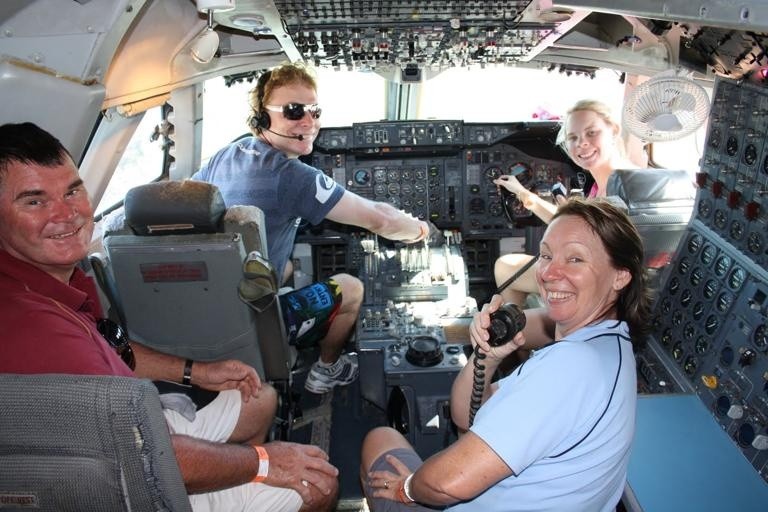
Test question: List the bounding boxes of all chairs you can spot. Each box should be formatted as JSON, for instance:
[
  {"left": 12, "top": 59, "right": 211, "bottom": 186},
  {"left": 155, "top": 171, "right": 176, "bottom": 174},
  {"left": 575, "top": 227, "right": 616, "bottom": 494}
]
[
  {"left": 526, "top": 169, "right": 698, "bottom": 308},
  {"left": 0, "top": 375, "right": 194, "bottom": 512},
  {"left": 103, "top": 181, "right": 298, "bottom": 388}
]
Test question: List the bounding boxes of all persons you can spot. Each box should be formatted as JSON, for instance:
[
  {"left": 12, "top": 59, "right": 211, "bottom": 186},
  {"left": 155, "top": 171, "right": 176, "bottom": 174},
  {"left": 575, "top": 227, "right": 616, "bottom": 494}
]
[
  {"left": 359, "top": 197, "right": 652, "bottom": 511},
  {"left": 493, "top": 99, "right": 643, "bottom": 365},
  {"left": 0, "top": 121, "right": 341, "bottom": 512},
  {"left": 187, "top": 66, "right": 427, "bottom": 395}
]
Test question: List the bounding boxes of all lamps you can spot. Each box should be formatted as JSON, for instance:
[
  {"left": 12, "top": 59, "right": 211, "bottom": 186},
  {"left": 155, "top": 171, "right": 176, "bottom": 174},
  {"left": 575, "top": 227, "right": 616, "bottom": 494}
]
[{"left": 190, "top": 0, "right": 236, "bottom": 63}]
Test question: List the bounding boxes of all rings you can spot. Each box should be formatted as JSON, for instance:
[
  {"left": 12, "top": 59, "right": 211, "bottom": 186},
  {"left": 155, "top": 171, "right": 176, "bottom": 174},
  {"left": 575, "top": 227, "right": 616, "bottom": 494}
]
[{"left": 384, "top": 482, "right": 387, "bottom": 490}]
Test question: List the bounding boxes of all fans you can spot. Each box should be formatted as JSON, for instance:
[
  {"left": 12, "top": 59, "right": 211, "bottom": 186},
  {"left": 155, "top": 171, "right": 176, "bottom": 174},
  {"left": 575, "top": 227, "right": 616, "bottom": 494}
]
[{"left": 622, "top": 77, "right": 711, "bottom": 142}]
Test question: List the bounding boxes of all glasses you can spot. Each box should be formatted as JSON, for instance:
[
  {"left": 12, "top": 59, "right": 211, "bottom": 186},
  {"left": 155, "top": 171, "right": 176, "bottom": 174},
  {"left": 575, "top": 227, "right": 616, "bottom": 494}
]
[
  {"left": 95, "top": 316, "right": 137, "bottom": 374},
  {"left": 263, "top": 102, "right": 323, "bottom": 121}
]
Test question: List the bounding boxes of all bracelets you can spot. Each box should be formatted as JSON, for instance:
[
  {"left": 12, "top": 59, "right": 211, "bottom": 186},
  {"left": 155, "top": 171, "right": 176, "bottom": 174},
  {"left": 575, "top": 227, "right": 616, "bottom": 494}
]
[
  {"left": 397, "top": 472, "right": 416, "bottom": 505},
  {"left": 253, "top": 444, "right": 269, "bottom": 485},
  {"left": 182, "top": 356, "right": 194, "bottom": 387}
]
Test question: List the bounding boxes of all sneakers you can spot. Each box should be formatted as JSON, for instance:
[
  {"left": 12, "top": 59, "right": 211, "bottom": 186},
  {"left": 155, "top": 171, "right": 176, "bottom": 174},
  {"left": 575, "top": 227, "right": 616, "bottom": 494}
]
[{"left": 304, "top": 350, "right": 362, "bottom": 396}]
